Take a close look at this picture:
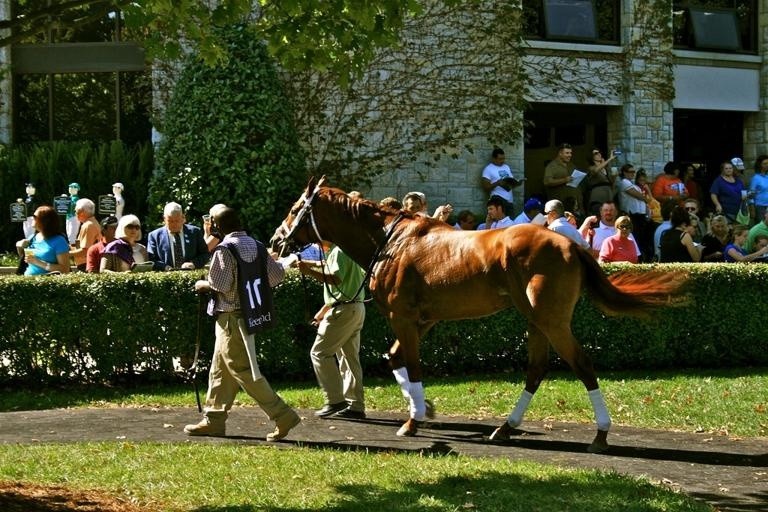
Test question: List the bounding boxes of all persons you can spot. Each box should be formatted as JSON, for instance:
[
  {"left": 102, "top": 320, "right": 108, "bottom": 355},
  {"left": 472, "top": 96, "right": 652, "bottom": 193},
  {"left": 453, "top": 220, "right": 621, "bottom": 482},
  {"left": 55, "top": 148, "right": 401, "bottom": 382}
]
[
  {"left": 297, "top": 245, "right": 367, "bottom": 420},
  {"left": 184, "top": 203, "right": 301, "bottom": 443}
]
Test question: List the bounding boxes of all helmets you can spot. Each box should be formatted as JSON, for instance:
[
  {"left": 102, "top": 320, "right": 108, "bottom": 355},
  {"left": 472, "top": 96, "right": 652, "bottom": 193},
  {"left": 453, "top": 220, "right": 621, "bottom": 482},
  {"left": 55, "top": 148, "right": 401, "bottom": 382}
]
[{"left": 209, "top": 203, "right": 241, "bottom": 232}]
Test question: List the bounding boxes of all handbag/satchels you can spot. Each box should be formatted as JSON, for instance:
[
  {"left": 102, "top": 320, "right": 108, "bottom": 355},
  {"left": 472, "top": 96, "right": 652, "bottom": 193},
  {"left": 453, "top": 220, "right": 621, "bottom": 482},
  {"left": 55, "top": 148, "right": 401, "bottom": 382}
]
[
  {"left": 16, "top": 246, "right": 32, "bottom": 276},
  {"left": 736, "top": 201, "right": 751, "bottom": 225}
]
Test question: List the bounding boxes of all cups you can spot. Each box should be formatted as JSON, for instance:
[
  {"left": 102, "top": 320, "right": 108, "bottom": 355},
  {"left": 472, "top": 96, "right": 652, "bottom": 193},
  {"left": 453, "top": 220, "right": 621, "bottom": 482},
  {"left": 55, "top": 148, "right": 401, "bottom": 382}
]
[
  {"left": 611, "top": 166, "right": 618, "bottom": 176},
  {"left": 202, "top": 213, "right": 211, "bottom": 223},
  {"left": 23, "top": 248, "right": 33, "bottom": 258},
  {"left": 679, "top": 183, "right": 684, "bottom": 194},
  {"left": 741, "top": 191, "right": 747, "bottom": 199}
]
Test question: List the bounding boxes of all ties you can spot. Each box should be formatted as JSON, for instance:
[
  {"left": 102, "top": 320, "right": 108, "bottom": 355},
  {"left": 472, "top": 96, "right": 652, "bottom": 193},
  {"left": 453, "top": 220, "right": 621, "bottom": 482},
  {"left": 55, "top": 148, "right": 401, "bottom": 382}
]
[{"left": 172, "top": 232, "right": 184, "bottom": 269}]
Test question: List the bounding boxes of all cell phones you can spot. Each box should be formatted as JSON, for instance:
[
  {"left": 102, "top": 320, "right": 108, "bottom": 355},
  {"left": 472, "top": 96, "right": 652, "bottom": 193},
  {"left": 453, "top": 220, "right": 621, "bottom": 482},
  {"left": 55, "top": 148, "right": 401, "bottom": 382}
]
[{"left": 613, "top": 151, "right": 622, "bottom": 155}]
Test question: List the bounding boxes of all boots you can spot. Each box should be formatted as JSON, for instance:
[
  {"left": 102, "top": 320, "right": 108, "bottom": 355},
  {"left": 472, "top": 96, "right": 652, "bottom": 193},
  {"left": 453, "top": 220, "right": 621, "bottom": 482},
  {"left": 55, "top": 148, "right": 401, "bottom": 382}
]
[
  {"left": 183, "top": 413, "right": 226, "bottom": 437},
  {"left": 266, "top": 412, "right": 301, "bottom": 442}
]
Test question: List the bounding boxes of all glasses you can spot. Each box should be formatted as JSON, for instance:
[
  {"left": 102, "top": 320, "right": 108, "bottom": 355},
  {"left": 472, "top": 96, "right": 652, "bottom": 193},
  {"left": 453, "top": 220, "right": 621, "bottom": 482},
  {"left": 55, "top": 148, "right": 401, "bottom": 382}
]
[
  {"left": 685, "top": 202, "right": 698, "bottom": 229},
  {"left": 618, "top": 224, "right": 632, "bottom": 228},
  {"left": 594, "top": 149, "right": 601, "bottom": 154},
  {"left": 626, "top": 169, "right": 634, "bottom": 173},
  {"left": 125, "top": 224, "right": 142, "bottom": 229},
  {"left": 541, "top": 210, "right": 556, "bottom": 214}
]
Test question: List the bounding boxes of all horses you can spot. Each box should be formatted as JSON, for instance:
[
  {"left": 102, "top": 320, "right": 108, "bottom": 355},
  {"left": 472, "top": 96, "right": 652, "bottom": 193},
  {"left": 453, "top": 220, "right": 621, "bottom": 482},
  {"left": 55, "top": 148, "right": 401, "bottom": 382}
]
[{"left": 269, "top": 174, "right": 694, "bottom": 454}]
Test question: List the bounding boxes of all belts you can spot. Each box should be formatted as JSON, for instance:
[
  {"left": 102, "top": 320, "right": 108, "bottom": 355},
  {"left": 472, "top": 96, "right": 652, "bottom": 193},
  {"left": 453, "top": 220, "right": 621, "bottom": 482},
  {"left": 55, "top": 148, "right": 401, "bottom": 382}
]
[{"left": 331, "top": 300, "right": 355, "bottom": 306}]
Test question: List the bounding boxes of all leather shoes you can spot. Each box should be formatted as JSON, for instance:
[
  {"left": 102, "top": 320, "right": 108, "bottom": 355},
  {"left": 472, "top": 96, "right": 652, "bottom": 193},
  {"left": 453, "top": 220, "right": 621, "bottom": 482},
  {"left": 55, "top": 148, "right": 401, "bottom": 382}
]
[
  {"left": 314, "top": 400, "right": 349, "bottom": 417},
  {"left": 334, "top": 405, "right": 366, "bottom": 419}
]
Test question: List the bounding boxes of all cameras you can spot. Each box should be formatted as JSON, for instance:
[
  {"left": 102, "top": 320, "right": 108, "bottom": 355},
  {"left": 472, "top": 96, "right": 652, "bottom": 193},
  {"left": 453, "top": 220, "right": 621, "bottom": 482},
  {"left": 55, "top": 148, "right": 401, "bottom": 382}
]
[{"left": 445, "top": 203, "right": 454, "bottom": 213}]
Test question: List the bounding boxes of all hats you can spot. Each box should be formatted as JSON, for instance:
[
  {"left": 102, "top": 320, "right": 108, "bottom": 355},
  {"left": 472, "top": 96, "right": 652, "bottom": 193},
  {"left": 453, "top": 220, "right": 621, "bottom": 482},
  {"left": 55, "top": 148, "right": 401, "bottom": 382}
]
[
  {"left": 731, "top": 157, "right": 744, "bottom": 170},
  {"left": 525, "top": 198, "right": 543, "bottom": 208},
  {"left": 18, "top": 179, "right": 125, "bottom": 191}
]
